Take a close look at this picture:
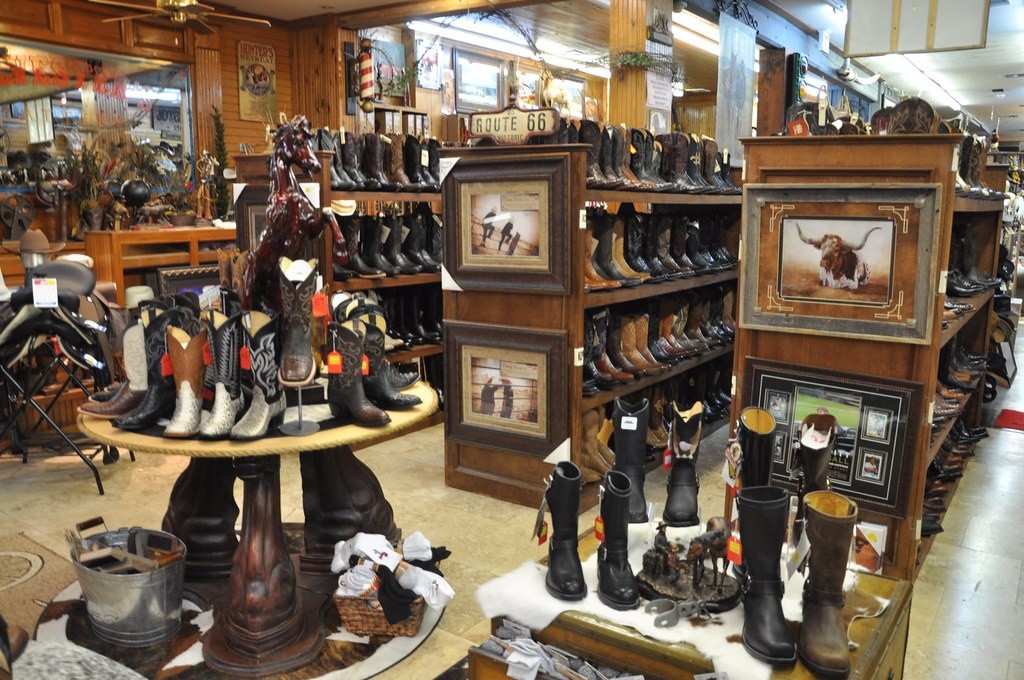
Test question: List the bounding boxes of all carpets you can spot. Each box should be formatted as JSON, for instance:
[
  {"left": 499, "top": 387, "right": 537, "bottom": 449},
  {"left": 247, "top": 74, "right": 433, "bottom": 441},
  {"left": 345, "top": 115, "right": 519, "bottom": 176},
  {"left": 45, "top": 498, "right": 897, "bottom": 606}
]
[
  {"left": 995, "top": 408, "right": 1024, "bottom": 431},
  {"left": 0, "top": 531, "right": 78, "bottom": 653}
]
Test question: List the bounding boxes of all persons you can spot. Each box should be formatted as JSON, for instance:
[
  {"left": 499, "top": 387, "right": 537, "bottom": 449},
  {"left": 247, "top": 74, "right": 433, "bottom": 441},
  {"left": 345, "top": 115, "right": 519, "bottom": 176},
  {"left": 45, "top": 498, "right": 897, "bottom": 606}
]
[
  {"left": 479, "top": 206, "right": 497, "bottom": 248},
  {"left": 500, "top": 376, "right": 513, "bottom": 418},
  {"left": 498, "top": 218, "right": 513, "bottom": 251},
  {"left": 864, "top": 456, "right": 878, "bottom": 478},
  {"left": 480, "top": 374, "right": 503, "bottom": 416}
]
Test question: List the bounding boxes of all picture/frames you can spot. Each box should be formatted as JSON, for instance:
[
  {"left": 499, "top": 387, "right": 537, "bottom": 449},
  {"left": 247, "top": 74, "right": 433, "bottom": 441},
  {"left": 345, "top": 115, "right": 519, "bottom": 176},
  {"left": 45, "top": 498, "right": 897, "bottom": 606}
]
[
  {"left": 739, "top": 178, "right": 943, "bottom": 346},
  {"left": 739, "top": 358, "right": 921, "bottom": 515},
  {"left": 452, "top": 47, "right": 587, "bottom": 121},
  {"left": 448, "top": 153, "right": 567, "bottom": 293},
  {"left": 441, "top": 322, "right": 569, "bottom": 459}
]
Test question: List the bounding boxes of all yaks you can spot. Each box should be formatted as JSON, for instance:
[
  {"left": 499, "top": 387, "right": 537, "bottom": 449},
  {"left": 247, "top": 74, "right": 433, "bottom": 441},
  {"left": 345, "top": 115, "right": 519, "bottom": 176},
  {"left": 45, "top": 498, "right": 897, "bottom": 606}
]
[{"left": 794, "top": 222, "right": 883, "bottom": 290}]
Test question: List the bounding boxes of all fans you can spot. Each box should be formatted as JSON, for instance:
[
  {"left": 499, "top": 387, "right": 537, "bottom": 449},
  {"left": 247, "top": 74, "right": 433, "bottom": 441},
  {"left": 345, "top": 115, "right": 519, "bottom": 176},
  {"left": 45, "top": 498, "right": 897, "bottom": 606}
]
[{"left": 92, "top": 0, "right": 272, "bottom": 34}]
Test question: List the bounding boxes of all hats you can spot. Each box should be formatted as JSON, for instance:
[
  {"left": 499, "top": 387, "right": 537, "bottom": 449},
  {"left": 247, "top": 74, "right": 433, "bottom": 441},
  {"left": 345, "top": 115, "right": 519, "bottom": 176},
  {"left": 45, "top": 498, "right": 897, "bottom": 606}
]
[{"left": 7, "top": 229, "right": 67, "bottom": 259}]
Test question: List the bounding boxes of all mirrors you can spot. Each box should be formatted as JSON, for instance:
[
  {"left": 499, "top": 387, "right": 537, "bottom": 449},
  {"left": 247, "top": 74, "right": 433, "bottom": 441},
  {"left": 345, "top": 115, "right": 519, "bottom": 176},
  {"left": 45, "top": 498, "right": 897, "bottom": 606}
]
[{"left": 0, "top": 35, "right": 199, "bottom": 196}]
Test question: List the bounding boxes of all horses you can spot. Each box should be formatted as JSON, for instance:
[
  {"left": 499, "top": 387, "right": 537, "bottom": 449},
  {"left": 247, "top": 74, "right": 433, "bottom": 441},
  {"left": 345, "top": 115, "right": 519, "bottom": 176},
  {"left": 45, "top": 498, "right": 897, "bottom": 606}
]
[
  {"left": 688, "top": 517, "right": 732, "bottom": 595},
  {"left": 237, "top": 111, "right": 348, "bottom": 313}
]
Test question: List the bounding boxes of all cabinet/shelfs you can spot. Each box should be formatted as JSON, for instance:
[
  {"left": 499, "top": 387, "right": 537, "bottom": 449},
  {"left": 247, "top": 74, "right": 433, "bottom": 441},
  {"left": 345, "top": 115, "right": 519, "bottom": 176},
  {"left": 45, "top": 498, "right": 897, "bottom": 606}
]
[
  {"left": 83, "top": 220, "right": 234, "bottom": 309},
  {"left": 735, "top": 137, "right": 1024, "bottom": 579},
  {"left": 440, "top": 146, "right": 744, "bottom": 513},
  {"left": 470, "top": 519, "right": 925, "bottom": 680},
  {"left": 233, "top": 150, "right": 449, "bottom": 421}
]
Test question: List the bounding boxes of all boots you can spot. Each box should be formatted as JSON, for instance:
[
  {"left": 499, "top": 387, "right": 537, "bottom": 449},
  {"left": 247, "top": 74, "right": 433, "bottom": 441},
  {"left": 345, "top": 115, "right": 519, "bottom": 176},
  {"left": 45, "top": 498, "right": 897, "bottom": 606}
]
[{"left": 71, "top": 52, "right": 991, "bottom": 678}]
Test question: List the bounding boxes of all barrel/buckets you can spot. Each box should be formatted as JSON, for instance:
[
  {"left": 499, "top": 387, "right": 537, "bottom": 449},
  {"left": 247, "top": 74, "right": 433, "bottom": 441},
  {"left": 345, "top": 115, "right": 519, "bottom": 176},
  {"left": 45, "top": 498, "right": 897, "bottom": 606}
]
[{"left": 69, "top": 515, "right": 188, "bottom": 649}]
